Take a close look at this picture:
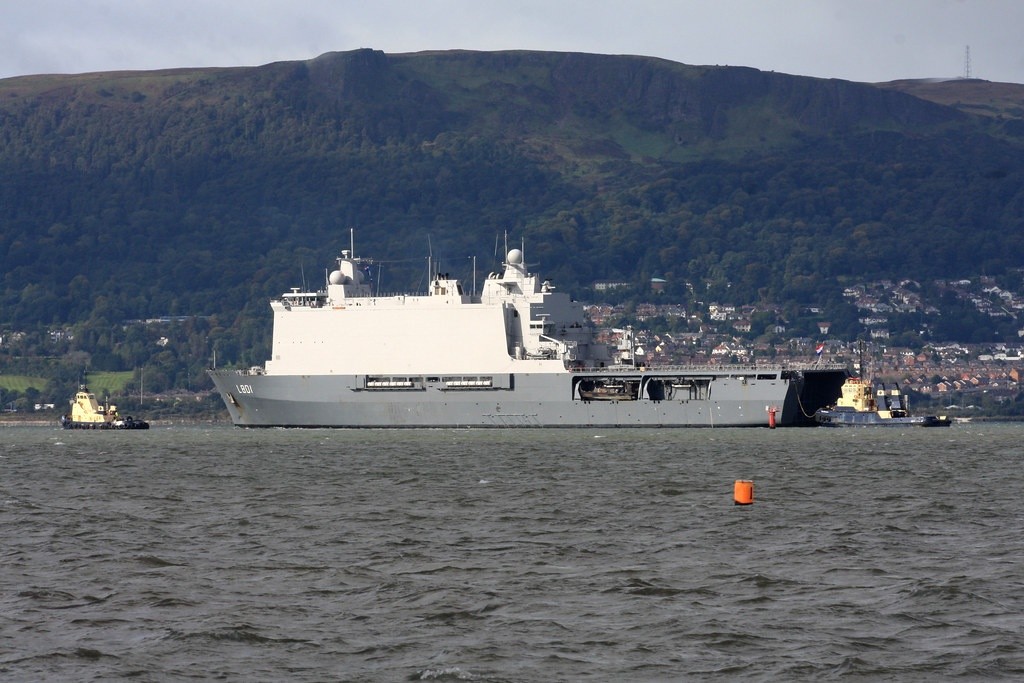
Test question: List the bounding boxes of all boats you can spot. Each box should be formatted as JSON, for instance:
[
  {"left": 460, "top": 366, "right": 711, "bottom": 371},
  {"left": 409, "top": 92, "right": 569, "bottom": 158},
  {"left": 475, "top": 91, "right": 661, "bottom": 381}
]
[
  {"left": 815, "top": 382, "right": 953, "bottom": 428},
  {"left": 59, "top": 365, "right": 150, "bottom": 430},
  {"left": 203, "top": 228, "right": 866, "bottom": 428}
]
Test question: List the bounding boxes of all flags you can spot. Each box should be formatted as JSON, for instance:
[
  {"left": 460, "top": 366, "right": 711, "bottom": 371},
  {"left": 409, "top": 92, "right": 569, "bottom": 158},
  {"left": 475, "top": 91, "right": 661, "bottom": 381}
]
[{"left": 815, "top": 341, "right": 826, "bottom": 356}]
